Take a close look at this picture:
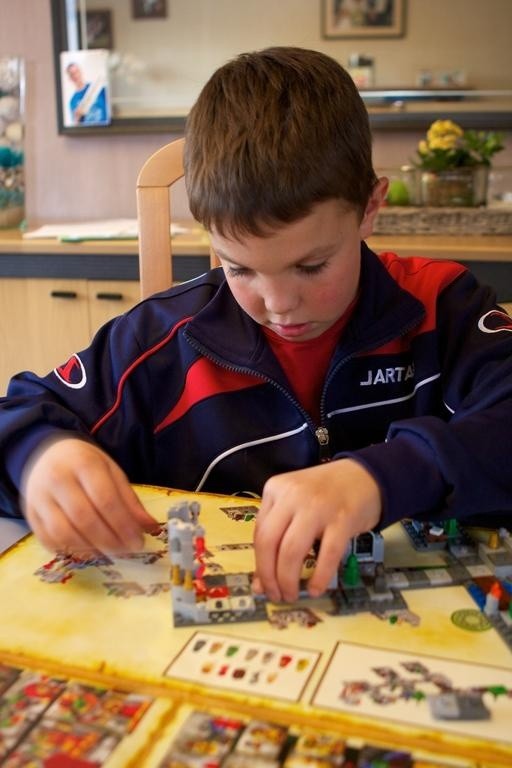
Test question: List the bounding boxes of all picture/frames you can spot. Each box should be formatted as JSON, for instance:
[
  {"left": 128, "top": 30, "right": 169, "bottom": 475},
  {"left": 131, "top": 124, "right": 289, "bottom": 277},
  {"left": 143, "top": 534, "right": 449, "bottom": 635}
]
[
  {"left": 77, "top": 1, "right": 167, "bottom": 55},
  {"left": 318, "top": 0, "right": 411, "bottom": 43}
]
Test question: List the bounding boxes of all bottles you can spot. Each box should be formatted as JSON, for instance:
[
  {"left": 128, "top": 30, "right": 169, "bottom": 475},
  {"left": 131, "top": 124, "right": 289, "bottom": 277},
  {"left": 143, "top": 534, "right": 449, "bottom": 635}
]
[
  {"left": 399, "top": 164, "right": 417, "bottom": 206},
  {"left": 347, "top": 52, "right": 374, "bottom": 91}
]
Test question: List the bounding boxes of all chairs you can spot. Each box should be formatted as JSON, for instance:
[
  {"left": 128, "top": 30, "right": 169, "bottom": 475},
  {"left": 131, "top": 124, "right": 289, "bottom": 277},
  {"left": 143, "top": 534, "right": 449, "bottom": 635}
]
[{"left": 135, "top": 137, "right": 222, "bottom": 306}]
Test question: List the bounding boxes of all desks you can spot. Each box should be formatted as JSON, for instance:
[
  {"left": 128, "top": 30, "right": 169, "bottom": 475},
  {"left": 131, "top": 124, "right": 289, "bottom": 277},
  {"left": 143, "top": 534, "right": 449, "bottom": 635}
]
[{"left": 0, "top": 481, "right": 512, "bottom": 766}]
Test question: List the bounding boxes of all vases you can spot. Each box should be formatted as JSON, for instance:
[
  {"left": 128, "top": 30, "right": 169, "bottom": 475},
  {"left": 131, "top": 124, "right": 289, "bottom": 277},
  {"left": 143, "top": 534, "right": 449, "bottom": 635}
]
[{"left": 420, "top": 171, "right": 487, "bottom": 207}]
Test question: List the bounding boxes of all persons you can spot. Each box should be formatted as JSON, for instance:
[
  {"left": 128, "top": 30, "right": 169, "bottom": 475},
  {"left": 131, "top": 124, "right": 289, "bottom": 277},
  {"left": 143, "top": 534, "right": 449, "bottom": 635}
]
[
  {"left": 66, "top": 63, "right": 108, "bottom": 126},
  {"left": 1, "top": 46, "right": 512, "bottom": 608}
]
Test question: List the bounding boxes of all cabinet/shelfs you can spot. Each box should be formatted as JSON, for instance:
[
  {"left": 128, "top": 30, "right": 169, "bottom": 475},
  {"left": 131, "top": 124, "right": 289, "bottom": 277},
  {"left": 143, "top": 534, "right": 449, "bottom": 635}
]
[{"left": 0, "top": 252, "right": 211, "bottom": 409}]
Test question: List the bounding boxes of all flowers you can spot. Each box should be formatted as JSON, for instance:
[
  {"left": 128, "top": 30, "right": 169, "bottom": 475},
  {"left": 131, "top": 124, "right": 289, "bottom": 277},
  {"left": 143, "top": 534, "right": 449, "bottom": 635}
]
[{"left": 400, "top": 121, "right": 503, "bottom": 171}]
